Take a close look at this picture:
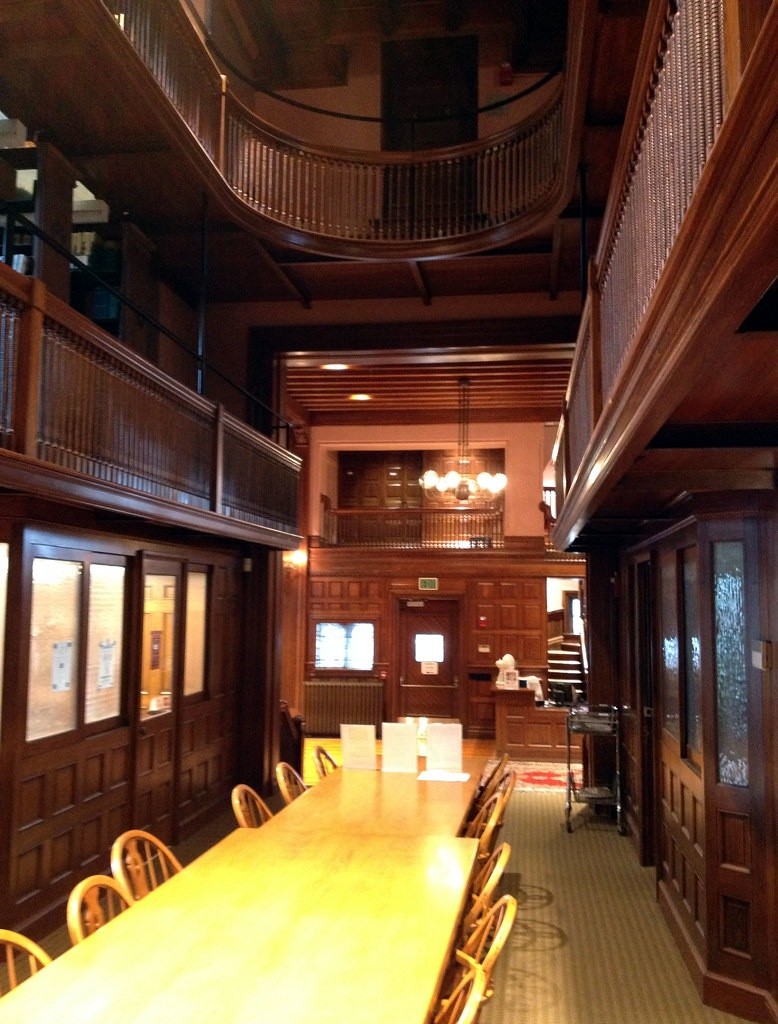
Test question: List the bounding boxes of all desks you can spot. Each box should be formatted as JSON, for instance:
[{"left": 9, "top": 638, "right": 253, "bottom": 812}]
[{"left": 0, "top": 755, "right": 490, "bottom": 1024}]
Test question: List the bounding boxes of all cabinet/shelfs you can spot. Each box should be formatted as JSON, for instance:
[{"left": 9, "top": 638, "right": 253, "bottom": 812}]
[{"left": 493, "top": 689, "right": 583, "bottom": 764}]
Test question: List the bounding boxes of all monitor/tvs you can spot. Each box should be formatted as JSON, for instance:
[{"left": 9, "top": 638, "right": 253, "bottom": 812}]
[{"left": 548, "top": 683, "right": 573, "bottom": 706}]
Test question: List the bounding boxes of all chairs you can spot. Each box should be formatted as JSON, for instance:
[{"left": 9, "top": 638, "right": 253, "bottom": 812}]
[{"left": 1, "top": 742, "right": 523, "bottom": 1023}]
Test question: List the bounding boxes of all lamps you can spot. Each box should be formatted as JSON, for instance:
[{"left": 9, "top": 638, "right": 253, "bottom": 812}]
[{"left": 417, "top": 374, "right": 508, "bottom": 501}]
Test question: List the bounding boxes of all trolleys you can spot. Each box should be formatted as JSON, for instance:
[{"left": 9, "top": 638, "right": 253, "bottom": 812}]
[{"left": 565, "top": 703, "right": 627, "bottom": 836}]
